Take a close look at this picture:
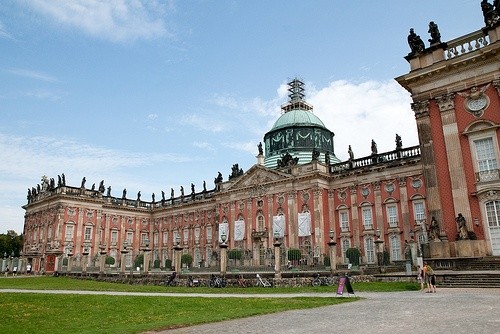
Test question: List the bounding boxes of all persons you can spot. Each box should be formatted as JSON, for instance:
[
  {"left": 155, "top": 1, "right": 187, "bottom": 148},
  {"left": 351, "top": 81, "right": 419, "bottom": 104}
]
[
  {"left": 13, "top": 265, "right": 18, "bottom": 276},
  {"left": 416, "top": 263, "right": 437, "bottom": 293},
  {"left": 429, "top": 215, "right": 442, "bottom": 243},
  {"left": 3, "top": 264, "right": 10, "bottom": 277},
  {"left": 26, "top": 262, "right": 32, "bottom": 274},
  {"left": 454, "top": 213, "right": 468, "bottom": 239}
]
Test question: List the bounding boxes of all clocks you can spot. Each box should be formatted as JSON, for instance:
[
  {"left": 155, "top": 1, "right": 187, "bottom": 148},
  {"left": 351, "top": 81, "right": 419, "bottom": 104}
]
[{"left": 466, "top": 96, "right": 487, "bottom": 111}]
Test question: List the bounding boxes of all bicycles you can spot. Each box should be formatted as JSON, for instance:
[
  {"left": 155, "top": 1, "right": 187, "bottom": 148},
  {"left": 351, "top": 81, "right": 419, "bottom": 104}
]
[
  {"left": 312, "top": 272, "right": 334, "bottom": 287},
  {"left": 186, "top": 275, "right": 208, "bottom": 287},
  {"left": 209, "top": 273, "right": 228, "bottom": 287}
]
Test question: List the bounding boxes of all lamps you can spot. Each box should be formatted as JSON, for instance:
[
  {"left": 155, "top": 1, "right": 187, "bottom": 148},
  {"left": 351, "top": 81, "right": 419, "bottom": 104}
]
[
  {"left": 123, "top": 240, "right": 128, "bottom": 250},
  {"left": 144, "top": 236, "right": 150, "bottom": 248},
  {"left": 84, "top": 243, "right": 88, "bottom": 251},
  {"left": 176, "top": 234, "right": 182, "bottom": 246},
  {"left": 329, "top": 230, "right": 335, "bottom": 243},
  {"left": 376, "top": 227, "right": 382, "bottom": 240},
  {"left": 68, "top": 244, "right": 73, "bottom": 253},
  {"left": 220, "top": 231, "right": 227, "bottom": 245},
  {"left": 101, "top": 242, "right": 106, "bottom": 251},
  {"left": 274, "top": 229, "right": 280, "bottom": 242}
]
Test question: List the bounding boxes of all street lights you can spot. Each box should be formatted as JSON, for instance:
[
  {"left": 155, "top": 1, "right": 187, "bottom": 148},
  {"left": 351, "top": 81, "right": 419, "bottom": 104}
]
[
  {"left": 120, "top": 242, "right": 130, "bottom": 274},
  {"left": 326, "top": 229, "right": 337, "bottom": 273},
  {"left": 410, "top": 229, "right": 417, "bottom": 276},
  {"left": 81, "top": 243, "right": 90, "bottom": 275},
  {"left": 1, "top": 252, "right": 7, "bottom": 273},
  {"left": 99, "top": 242, "right": 107, "bottom": 274},
  {"left": 173, "top": 233, "right": 184, "bottom": 274},
  {"left": 143, "top": 237, "right": 152, "bottom": 275},
  {"left": 374, "top": 228, "right": 385, "bottom": 275},
  {"left": 67, "top": 244, "right": 74, "bottom": 273},
  {"left": 273, "top": 228, "right": 282, "bottom": 274},
  {"left": 219, "top": 232, "right": 228, "bottom": 272}
]
